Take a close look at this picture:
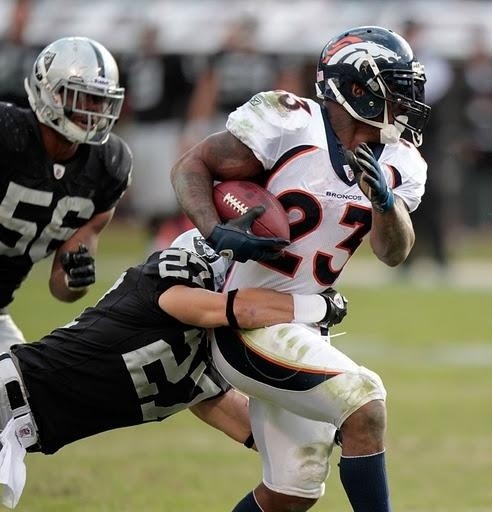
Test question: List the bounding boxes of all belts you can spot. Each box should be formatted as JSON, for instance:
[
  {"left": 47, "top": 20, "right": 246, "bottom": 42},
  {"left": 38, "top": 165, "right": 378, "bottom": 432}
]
[{"left": 1, "top": 353, "right": 41, "bottom": 452}]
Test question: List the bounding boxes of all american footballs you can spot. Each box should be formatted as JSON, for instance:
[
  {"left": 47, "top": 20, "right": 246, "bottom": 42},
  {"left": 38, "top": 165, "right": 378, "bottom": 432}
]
[{"left": 213, "top": 181, "right": 290, "bottom": 240}]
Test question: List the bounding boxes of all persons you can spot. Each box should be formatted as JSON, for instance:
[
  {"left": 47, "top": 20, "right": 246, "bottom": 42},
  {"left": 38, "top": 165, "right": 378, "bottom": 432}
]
[
  {"left": 179, "top": 12, "right": 306, "bottom": 157},
  {"left": 394, "top": 10, "right": 451, "bottom": 282},
  {"left": 167, "top": 24, "right": 428, "bottom": 512},
  {"left": 0, "top": 1, "right": 46, "bottom": 106},
  {"left": 0, "top": 222, "right": 348, "bottom": 509},
  {"left": 114, "top": 18, "right": 195, "bottom": 239},
  {"left": 0, "top": 36, "right": 134, "bottom": 353},
  {"left": 446, "top": 19, "right": 491, "bottom": 237}
]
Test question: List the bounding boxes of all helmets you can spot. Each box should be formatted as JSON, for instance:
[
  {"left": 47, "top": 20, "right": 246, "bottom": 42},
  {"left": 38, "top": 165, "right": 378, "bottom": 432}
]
[
  {"left": 25, "top": 37, "right": 124, "bottom": 145},
  {"left": 170, "top": 227, "right": 235, "bottom": 292},
  {"left": 316, "top": 26, "right": 430, "bottom": 145}
]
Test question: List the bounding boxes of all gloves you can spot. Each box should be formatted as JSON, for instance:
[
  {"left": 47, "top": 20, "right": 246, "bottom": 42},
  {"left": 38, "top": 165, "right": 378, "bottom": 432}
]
[
  {"left": 205, "top": 207, "right": 290, "bottom": 262},
  {"left": 60, "top": 244, "right": 95, "bottom": 291},
  {"left": 343, "top": 142, "right": 394, "bottom": 214},
  {"left": 317, "top": 287, "right": 349, "bottom": 330}
]
